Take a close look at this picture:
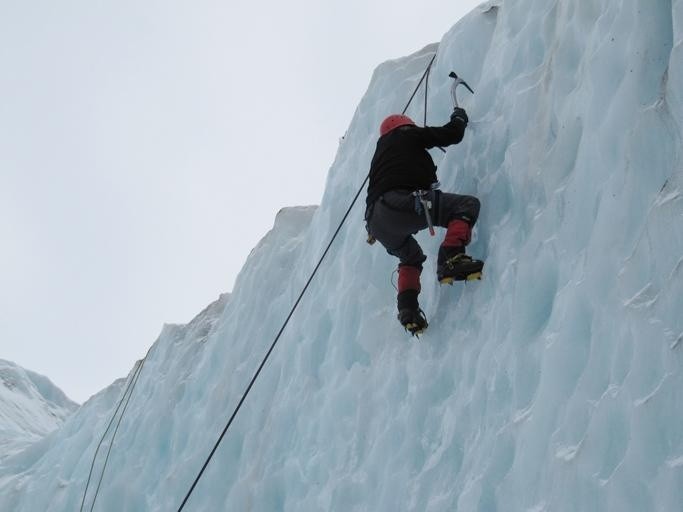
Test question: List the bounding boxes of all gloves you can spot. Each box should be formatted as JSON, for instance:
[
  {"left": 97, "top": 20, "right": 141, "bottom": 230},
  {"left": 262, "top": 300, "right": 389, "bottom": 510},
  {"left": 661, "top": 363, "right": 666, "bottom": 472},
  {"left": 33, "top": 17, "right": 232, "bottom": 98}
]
[{"left": 451, "top": 107, "right": 468, "bottom": 123}]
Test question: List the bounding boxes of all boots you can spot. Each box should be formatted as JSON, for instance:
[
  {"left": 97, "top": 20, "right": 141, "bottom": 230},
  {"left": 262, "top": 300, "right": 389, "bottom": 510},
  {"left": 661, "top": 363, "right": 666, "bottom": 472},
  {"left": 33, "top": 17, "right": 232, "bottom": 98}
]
[
  {"left": 437, "top": 245, "right": 483, "bottom": 286},
  {"left": 396, "top": 288, "right": 429, "bottom": 336}
]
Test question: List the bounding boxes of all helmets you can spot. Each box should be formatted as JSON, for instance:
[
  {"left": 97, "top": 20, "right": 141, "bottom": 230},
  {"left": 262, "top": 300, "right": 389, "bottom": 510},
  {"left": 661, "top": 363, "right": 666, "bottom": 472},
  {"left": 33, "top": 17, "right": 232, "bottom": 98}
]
[{"left": 380, "top": 114, "right": 415, "bottom": 136}]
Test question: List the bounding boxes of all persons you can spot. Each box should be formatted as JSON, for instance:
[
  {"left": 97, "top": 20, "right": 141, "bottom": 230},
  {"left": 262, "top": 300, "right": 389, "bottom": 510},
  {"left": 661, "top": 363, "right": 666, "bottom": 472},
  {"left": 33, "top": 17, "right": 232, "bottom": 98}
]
[{"left": 363, "top": 106, "right": 484, "bottom": 339}]
